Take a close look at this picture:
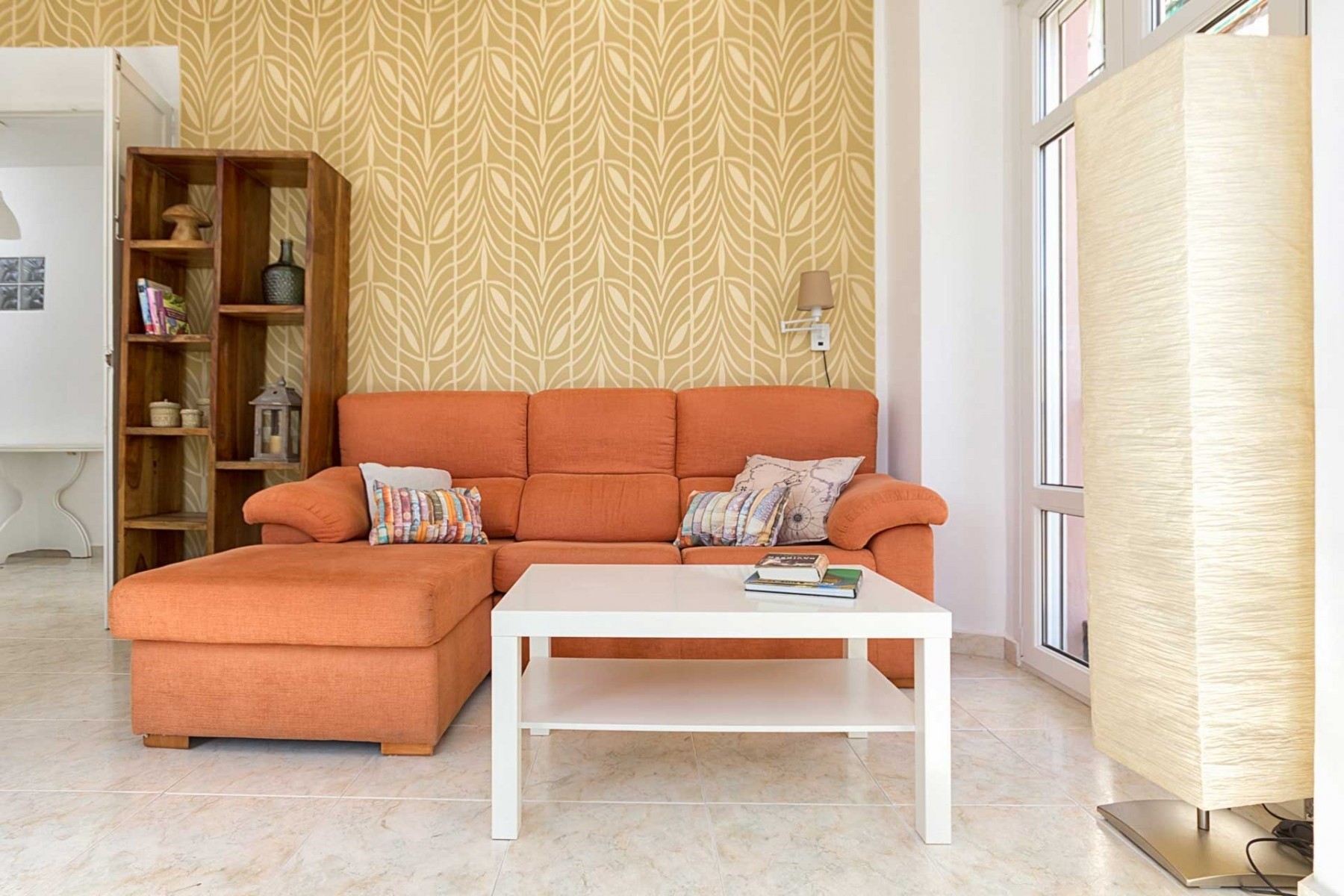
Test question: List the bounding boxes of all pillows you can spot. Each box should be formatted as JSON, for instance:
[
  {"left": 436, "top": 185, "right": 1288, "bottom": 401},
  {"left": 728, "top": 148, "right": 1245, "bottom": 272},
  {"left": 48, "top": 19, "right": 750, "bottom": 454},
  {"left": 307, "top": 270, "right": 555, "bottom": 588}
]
[
  {"left": 368, "top": 480, "right": 489, "bottom": 547},
  {"left": 673, "top": 485, "right": 791, "bottom": 548},
  {"left": 731, "top": 454, "right": 866, "bottom": 543},
  {"left": 358, "top": 462, "right": 453, "bottom": 524}
]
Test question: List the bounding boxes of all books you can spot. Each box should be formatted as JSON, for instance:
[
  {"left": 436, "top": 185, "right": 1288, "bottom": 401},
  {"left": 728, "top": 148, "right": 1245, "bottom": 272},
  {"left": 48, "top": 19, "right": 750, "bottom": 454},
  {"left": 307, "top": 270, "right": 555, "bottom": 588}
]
[
  {"left": 743, "top": 568, "right": 864, "bottom": 600},
  {"left": 752, "top": 551, "right": 830, "bottom": 583}
]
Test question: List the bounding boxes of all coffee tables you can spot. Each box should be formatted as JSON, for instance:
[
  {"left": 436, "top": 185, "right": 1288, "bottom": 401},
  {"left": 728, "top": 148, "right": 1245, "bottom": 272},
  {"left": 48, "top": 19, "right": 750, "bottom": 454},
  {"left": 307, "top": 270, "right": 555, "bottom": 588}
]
[{"left": 490, "top": 564, "right": 952, "bottom": 844}]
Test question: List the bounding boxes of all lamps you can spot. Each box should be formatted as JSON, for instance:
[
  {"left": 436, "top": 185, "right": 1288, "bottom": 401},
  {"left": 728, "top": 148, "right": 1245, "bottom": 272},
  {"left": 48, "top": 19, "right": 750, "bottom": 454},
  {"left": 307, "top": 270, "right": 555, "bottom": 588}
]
[
  {"left": 780, "top": 269, "right": 834, "bottom": 351},
  {"left": 1, "top": 190, "right": 21, "bottom": 239},
  {"left": 1073, "top": 32, "right": 1313, "bottom": 890}
]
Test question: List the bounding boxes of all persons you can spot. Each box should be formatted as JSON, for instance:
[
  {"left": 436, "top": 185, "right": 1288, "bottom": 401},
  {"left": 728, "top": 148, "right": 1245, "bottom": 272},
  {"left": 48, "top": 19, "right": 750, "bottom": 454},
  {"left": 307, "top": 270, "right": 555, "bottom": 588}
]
[{"left": 135, "top": 278, "right": 192, "bottom": 336}]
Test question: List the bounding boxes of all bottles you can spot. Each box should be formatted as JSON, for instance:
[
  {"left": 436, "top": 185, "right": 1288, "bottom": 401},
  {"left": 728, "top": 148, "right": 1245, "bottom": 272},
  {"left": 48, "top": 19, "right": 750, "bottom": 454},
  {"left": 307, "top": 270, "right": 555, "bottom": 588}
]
[{"left": 260, "top": 238, "right": 304, "bottom": 305}]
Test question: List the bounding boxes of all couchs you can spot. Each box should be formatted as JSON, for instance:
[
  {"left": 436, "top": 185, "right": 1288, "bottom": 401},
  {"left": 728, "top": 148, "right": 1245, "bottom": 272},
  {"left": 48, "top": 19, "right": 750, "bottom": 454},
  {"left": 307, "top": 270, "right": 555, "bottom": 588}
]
[{"left": 111, "top": 384, "right": 947, "bottom": 758}]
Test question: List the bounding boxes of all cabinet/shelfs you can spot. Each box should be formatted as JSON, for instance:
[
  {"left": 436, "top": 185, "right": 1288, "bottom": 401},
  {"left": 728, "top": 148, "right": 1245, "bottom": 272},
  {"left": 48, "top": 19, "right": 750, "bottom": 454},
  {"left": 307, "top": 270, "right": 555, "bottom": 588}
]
[{"left": 117, "top": 145, "right": 352, "bottom": 578}]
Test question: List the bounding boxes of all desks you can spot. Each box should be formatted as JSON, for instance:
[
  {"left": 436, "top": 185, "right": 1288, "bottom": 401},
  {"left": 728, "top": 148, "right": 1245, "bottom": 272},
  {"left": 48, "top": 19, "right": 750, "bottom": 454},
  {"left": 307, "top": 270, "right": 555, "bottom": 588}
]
[{"left": 0, "top": 443, "right": 105, "bottom": 564}]
